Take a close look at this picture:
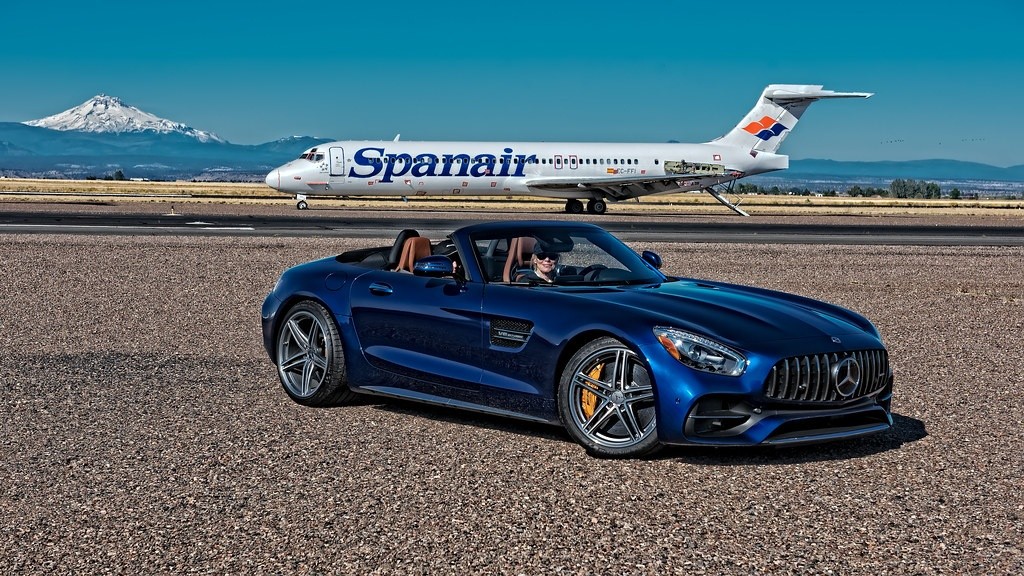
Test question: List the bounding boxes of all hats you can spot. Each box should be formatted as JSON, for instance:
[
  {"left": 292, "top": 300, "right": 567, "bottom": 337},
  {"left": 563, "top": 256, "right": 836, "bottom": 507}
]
[{"left": 534, "top": 241, "right": 555, "bottom": 254}]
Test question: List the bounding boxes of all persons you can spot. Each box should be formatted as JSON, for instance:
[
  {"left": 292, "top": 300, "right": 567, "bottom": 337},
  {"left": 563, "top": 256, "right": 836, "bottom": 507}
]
[
  {"left": 518, "top": 240, "right": 560, "bottom": 285},
  {"left": 432, "top": 239, "right": 463, "bottom": 278}
]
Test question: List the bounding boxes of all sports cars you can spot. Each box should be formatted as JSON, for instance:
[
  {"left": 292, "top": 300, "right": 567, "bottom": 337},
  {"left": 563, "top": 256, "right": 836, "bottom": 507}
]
[{"left": 261, "top": 220, "right": 895, "bottom": 460}]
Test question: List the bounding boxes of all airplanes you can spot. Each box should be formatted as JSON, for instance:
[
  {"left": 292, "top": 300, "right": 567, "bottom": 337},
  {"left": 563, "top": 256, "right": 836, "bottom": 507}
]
[{"left": 265, "top": 85, "right": 875, "bottom": 212}]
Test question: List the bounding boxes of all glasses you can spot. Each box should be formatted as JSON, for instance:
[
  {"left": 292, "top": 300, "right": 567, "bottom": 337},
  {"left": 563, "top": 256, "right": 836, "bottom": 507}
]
[{"left": 535, "top": 253, "right": 558, "bottom": 260}]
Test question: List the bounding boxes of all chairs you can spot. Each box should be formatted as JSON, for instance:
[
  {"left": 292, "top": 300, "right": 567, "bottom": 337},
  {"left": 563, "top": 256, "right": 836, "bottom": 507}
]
[
  {"left": 388, "top": 228, "right": 421, "bottom": 263},
  {"left": 394, "top": 237, "right": 432, "bottom": 273},
  {"left": 502, "top": 237, "right": 538, "bottom": 282}
]
